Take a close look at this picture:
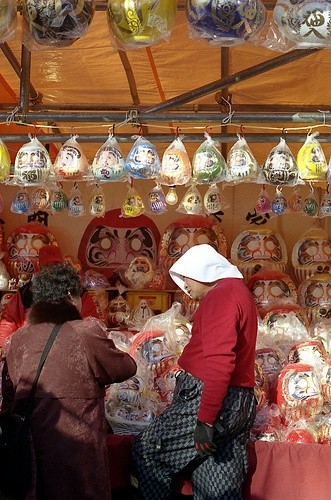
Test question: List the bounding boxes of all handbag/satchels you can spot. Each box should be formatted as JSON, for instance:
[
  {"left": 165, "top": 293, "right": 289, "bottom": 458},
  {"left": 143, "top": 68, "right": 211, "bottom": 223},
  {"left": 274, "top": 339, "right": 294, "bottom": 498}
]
[{"left": 1, "top": 420, "right": 32, "bottom": 500}]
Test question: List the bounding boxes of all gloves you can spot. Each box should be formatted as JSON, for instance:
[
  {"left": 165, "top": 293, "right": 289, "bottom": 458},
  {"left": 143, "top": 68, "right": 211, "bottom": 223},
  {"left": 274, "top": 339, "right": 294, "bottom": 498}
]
[{"left": 194, "top": 424, "right": 217, "bottom": 457}]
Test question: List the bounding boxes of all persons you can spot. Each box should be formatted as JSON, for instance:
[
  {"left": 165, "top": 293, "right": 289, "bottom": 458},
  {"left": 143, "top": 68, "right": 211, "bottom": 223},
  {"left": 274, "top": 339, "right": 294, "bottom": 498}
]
[
  {"left": 7, "top": 263, "right": 138, "bottom": 500},
  {"left": 0, "top": 246, "right": 99, "bottom": 360},
  {"left": 129, "top": 244, "right": 258, "bottom": 500}
]
